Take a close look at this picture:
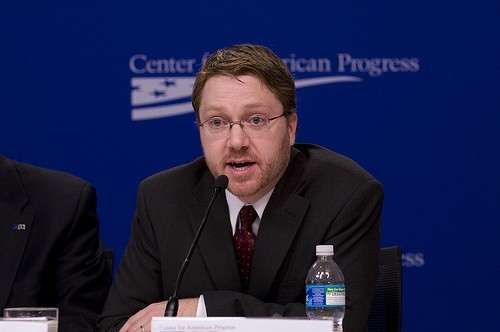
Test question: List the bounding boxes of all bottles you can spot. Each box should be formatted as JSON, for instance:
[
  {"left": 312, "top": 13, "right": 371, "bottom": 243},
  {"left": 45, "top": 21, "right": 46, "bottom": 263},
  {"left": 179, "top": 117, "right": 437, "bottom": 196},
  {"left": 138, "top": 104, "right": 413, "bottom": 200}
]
[{"left": 305, "top": 245, "right": 346, "bottom": 332}]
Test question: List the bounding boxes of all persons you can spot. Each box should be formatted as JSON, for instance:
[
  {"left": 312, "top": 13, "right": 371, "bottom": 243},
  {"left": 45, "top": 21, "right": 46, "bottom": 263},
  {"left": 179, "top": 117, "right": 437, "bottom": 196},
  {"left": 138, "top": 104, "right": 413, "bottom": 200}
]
[
  {"left": 0, "top": 157, "right": 108, "bottom": 332},
  {"left": 96, "top": 45, "right": 384, "bottom": 332}
]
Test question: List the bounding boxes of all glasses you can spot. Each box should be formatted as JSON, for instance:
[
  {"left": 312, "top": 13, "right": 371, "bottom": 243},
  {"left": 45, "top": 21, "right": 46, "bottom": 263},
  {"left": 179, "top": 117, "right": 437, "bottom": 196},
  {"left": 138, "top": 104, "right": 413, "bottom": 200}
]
[{"left": 197, "top": 110, "right": 289, "bottom": 132}]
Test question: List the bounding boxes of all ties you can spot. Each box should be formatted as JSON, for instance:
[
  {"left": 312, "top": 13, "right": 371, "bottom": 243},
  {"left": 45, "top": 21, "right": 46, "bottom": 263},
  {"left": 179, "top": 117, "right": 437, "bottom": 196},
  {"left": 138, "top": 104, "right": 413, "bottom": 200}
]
[{"left": 231, "top": 204, "right": 258, "bottom": 281}]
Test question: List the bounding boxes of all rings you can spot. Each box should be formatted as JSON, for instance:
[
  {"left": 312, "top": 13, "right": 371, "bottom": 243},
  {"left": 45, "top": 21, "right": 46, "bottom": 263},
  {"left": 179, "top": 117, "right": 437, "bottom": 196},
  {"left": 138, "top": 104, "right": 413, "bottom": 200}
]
[{"left": 139, "top": 324, "right": 146, "bottom": 332}]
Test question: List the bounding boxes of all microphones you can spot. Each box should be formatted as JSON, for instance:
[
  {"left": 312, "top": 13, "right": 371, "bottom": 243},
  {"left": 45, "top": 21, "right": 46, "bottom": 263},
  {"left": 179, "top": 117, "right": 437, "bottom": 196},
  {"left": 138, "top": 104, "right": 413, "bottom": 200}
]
[{"left": 164, "top": 175, "right": 229, "bottom": 317}]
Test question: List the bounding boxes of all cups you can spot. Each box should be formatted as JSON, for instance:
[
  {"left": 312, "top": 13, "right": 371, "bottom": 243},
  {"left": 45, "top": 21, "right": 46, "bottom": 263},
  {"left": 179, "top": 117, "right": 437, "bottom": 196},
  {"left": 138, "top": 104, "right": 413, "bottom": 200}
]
[{"left": 4, "top": 308, "right": 58, "bottom": 332}]
